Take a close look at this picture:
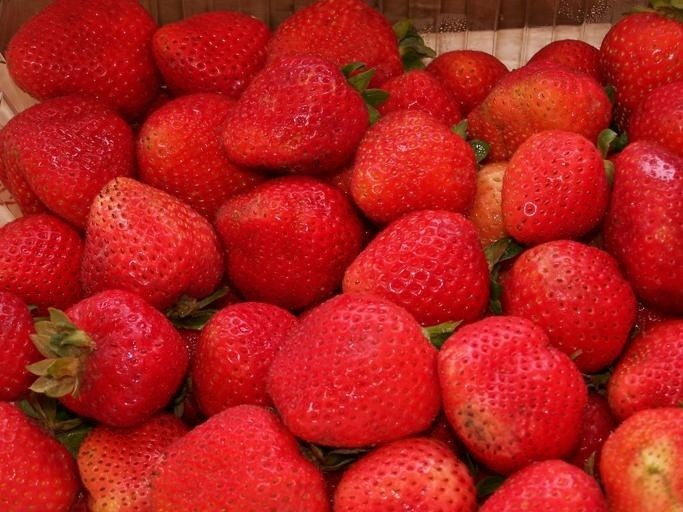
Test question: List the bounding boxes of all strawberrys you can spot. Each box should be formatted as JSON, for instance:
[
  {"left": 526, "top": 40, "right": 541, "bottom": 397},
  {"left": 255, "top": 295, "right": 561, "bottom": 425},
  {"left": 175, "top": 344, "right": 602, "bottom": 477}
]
[{"left": 1, "top": 0, "right": 681, "bottom": 512}]
